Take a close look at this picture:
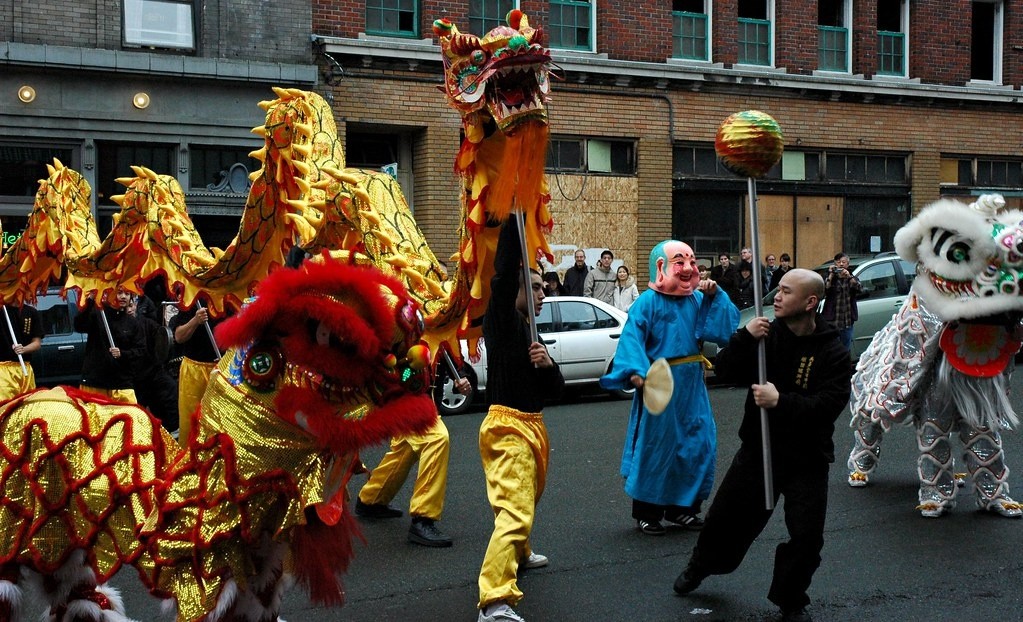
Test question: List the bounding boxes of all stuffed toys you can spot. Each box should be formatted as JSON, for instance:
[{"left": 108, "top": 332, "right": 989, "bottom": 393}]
[
  {"left": 0, "top": 263, "right": 431, "bottom": 622},
  {"left": 848, "top": 193, "right": 1023, "bottom": 520}
]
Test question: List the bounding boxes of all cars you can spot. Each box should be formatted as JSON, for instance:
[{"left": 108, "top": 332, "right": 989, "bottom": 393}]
[
  {"left": 433, "top": 296, "right": 640, "bottom": 416},
  {"left": 25, "top": 285, "right": 91, "bottom": 388}
]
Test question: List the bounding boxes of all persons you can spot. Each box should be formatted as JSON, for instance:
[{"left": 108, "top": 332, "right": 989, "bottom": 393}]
[
  {"left": 537, "top": 248, "right": 795, "bottom": 320},
  {"left": 674, "top": 269, "right": 852, "bottom": 622},
  {"left": 477, "top": 202, "right": 567, "bottom": 622},
  {"left": 822, "top": 253, "right": 862, "bottom": 358},
  {"left": 0, "top": 264, "right": 472, "bottom": 546},
  {"left": 601, "top": 240, "right": 740, "bottom": 535}
]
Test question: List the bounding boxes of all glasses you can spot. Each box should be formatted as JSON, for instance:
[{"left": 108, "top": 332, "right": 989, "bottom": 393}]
[{"left": 602, "top": 257, "right": 612, "bottom": 260}]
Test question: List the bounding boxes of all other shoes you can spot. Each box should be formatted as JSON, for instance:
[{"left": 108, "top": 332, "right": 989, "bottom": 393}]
[
  {"left": 170, "top": 428, "right": 181, "bottom": 438},
  {"left": 673, "top": 565, "right": 702, "bottom": 594},
  {"left": 780, "top": 604, "right": 812, "bottom": 622}
]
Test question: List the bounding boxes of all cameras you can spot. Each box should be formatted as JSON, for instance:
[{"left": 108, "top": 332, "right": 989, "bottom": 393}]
[{"left": 832, "top": 268, "right": 843, "bottom": 273}]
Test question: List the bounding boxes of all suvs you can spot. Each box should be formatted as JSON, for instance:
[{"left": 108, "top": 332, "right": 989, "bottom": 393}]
[{"left": 734, "top": 251, "right": 916, "bottom": 366}]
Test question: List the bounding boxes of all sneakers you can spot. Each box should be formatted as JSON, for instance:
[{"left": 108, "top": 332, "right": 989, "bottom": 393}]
[
  {"left": 518, "top": 548, "right": 549, "bottom": 567},
  {"left": 664, "top": 510, "right": 704, "bottom": 527},
  {"left": 637, "top": 517, "right": 665, "bottom": 535},
  {"left": 408, "top": 515, "right": 453, "bottom": 547},
  {"left": 355, "top": 496, "right": 404, "bottom": 520},
  {"left": 477, "top": 601, "right": 525, "bottom": 622}
]
